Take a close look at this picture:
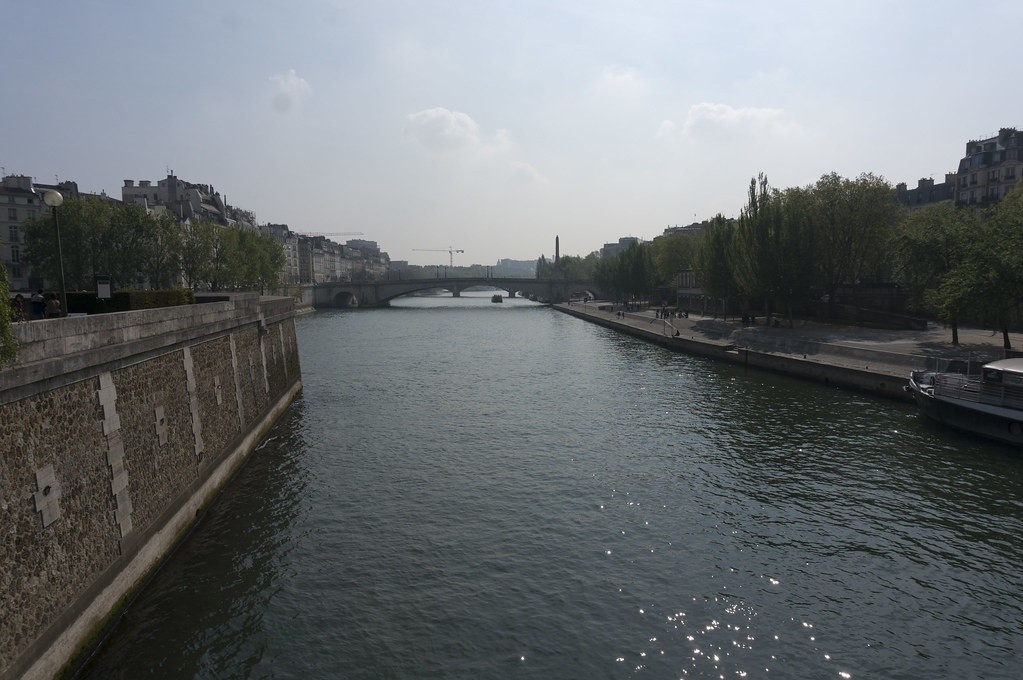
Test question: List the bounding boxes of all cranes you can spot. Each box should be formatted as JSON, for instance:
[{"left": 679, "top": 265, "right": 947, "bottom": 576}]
[{"left": 412, "top": 245, "right": 464, "bottom": 267}]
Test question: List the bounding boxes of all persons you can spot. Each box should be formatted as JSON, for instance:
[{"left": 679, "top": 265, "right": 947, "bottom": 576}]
[
  {"left": 10, "top": 288, "right": 61, "bottom": 320},
  {"left": 674, "top": 330, "right": 680, "bottom": 336},
  {"left": 618, "top": 311, "right": 621, "bottom": 319},
  {"left": 655, "top": 306, "right": 688, "bottom": 319},
  {"left": 622, "top": 311, "right": 624, "bottom": 319}
]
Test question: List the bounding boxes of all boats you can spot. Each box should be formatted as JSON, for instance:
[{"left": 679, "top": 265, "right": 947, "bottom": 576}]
[
  {"left": 491, "top": 295, "right": 503, "bottom": 303},
  {"left": 902, "top": 355, "right": 1023, "bottom": 445},
  {"left": 528, "top": 294, "right": 537, "bottom": 301}
]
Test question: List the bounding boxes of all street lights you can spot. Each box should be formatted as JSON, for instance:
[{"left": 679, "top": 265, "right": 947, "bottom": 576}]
[{"left": 43, "top": 189, "right": 69, "bottom": 315}]
